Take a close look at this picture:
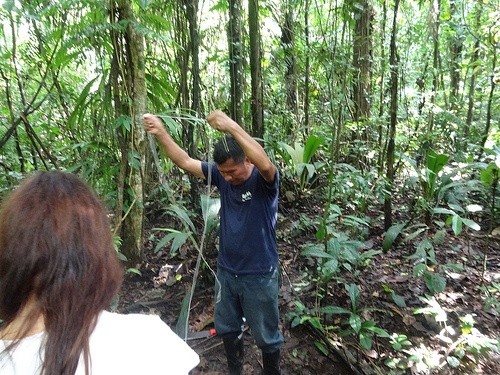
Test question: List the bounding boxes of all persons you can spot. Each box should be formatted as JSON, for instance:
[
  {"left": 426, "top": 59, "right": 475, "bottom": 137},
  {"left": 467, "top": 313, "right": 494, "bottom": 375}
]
[
  {"left": 0, "top": 171, "right": 201, "bottom": 375},
  {"left": 141, "top": 110, "right": 287, "bottom": 375}
]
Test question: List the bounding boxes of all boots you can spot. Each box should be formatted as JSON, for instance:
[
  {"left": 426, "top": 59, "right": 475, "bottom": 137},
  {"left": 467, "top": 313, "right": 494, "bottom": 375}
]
[
  {"left": 219, "top": 332, "right": 245, "bottom": 375},
  {"left": 261, "top": 348, "right": 283, "bottom": 375}
]
